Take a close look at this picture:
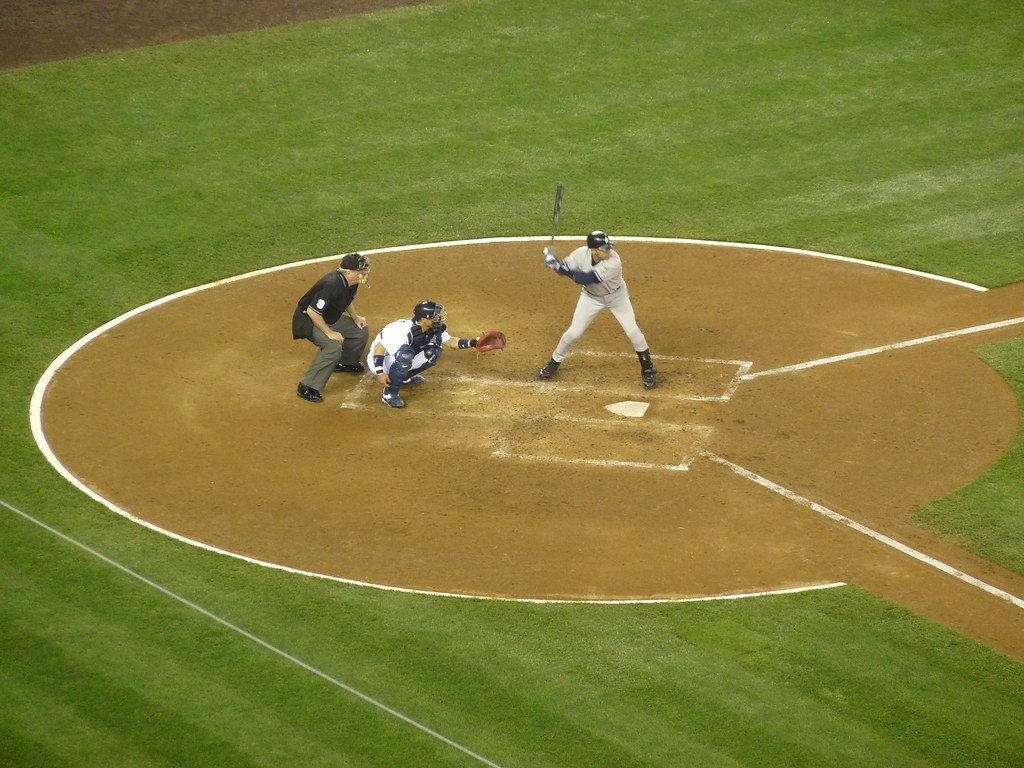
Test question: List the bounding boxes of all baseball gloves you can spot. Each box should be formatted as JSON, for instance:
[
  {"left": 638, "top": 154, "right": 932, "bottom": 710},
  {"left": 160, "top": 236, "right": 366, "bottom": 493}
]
[{"left": 475, "top": 331, "right": 507, "bottom": 353}]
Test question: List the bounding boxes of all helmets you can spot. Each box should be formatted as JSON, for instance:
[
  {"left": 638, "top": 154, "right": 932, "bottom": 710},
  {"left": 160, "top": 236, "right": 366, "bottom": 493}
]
[
  {"left": 340, "top": 253, "right": 371, "bottom": 287},
  {"left": 587, "top": 230, "right": 615, "bottom": 252},
  {"left": 414, "top": 300, "right": 446, "bottom": 332}
]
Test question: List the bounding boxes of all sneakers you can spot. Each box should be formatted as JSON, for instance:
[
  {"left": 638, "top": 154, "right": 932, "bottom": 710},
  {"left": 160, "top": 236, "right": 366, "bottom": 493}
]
[
  {"left": 539, "top": 361, "right": 559, "bottom": 379},
  {"left": 641, "top": 367, "right": 656, "bottom": 388},
  {"left": 382, "top": 393, "right": 404, "bottom": 408},
  {"left": 333, "top": 362, "right": 365, "bottom": 373},
  {"left": 297, "top": 382, "right": 321, "bottom": 401},
  {"left": 402, "top": 375, "right": 425, "bottom": 385}
]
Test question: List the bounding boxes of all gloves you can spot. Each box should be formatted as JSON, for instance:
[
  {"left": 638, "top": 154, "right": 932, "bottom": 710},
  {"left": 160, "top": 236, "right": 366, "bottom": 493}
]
[
  {"left": 545, "top": 255, "right": 560, "bottom": 271},
  {"left": 543, "top": 246, "right": 555, "bottom": 257}
]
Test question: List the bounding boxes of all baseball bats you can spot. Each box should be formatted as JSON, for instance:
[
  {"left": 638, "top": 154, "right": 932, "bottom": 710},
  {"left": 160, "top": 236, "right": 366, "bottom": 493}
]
[{"left": 548, "top": 182, "right": 564, "bottom": 250}]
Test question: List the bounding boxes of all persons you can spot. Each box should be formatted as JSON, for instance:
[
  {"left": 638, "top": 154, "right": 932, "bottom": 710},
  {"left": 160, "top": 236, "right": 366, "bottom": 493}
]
[
  {"left": 292, "top": 254, "right": 369, "bottom": 403},
  {"left": 366, "top": 300, "right": 481, "bottom": 408},
  {"left": 538, "top": 231, "right": 656, "bottom": 390}
]
